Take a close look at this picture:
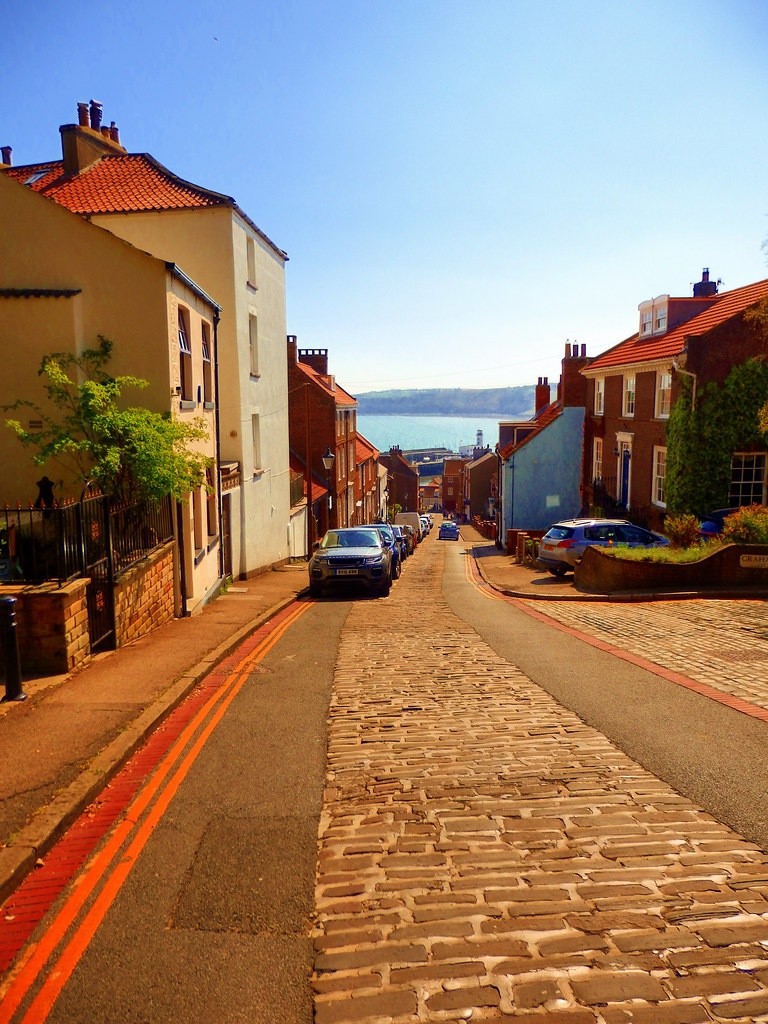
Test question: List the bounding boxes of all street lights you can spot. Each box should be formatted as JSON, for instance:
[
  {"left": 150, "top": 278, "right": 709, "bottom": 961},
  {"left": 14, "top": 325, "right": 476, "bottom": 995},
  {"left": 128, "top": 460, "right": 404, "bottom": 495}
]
[
  {"left": 384, "top": 486, "right": 389, "bottom": 523},
  {"left": 322, "top": 448, "right": 335, "bottom": 529}
]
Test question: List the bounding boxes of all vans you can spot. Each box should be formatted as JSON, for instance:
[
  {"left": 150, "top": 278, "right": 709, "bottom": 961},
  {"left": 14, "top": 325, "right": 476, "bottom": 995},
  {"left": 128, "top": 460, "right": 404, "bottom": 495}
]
[{"left": 394, "top": 512, "right": 422, "bottom": 542}]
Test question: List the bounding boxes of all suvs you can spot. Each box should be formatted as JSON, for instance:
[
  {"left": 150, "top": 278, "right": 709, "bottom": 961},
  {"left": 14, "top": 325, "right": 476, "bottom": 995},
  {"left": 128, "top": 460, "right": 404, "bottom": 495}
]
[
  {"left": 691, "top": 507, "right": 740, "bottom": 543},
  {"left": 537, "top": 518, "right": 669, "bottom": 575}
]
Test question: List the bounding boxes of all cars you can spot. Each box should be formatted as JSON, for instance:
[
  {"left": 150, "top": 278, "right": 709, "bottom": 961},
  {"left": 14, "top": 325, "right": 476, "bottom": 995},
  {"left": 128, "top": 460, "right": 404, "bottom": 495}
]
[
  {"left": 438, "top": 523, "right": 460, "bottom": 541},
  {"left": 308, "top": 528, "right": 392, "bottom": 597},
  {"left": 360, "top": 525, "right": 403, "bottom": 579},
  {"left": 419, "top": 515, "right": 433, "bottom": 537},
  {"left": 391, "top": 525, "right": 417, "bottom": 562}
]
